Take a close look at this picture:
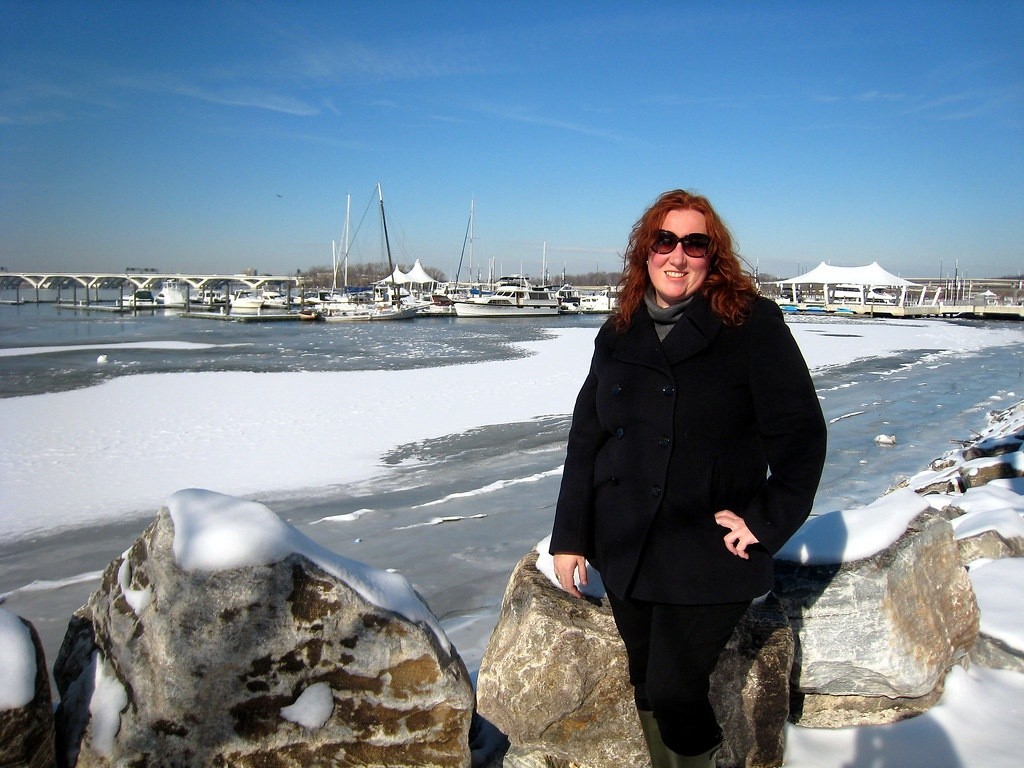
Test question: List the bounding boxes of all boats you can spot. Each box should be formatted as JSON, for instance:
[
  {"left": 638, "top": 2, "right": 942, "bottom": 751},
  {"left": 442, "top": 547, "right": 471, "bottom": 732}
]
[
  {"left": 115, "top": 182, "right": 626, "bottom": 319},
  {"left": 828, "top": 283, "right": 897, "bottom": 302}
]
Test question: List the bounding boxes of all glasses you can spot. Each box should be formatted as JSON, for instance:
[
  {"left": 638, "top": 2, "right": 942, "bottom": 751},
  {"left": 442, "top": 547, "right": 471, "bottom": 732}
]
[{"left": 650, "top": 228, "right": 715, "bottom": 258}]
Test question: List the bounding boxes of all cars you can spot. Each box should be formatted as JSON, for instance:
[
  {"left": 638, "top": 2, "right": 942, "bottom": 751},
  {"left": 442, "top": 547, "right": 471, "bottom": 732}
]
[{"left": 781, "top": 288, "right": 803, "bottom": 304}]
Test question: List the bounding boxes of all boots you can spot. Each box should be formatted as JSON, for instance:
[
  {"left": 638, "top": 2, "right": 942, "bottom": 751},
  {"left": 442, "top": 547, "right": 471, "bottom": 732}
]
[{"left": 637, "top": 709, "right": 737, "bottom": 768}]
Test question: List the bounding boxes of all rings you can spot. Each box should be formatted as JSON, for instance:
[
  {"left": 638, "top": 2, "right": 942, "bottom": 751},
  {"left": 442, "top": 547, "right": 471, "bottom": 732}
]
[{"left": 556, "top": 576, "right": 560, "bottom": 579}]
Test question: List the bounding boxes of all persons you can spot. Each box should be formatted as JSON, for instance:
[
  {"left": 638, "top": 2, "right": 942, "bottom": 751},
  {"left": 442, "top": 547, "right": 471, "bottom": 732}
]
[{"left": 549, "top": 190, "right": 827, "bottom": 768}]
